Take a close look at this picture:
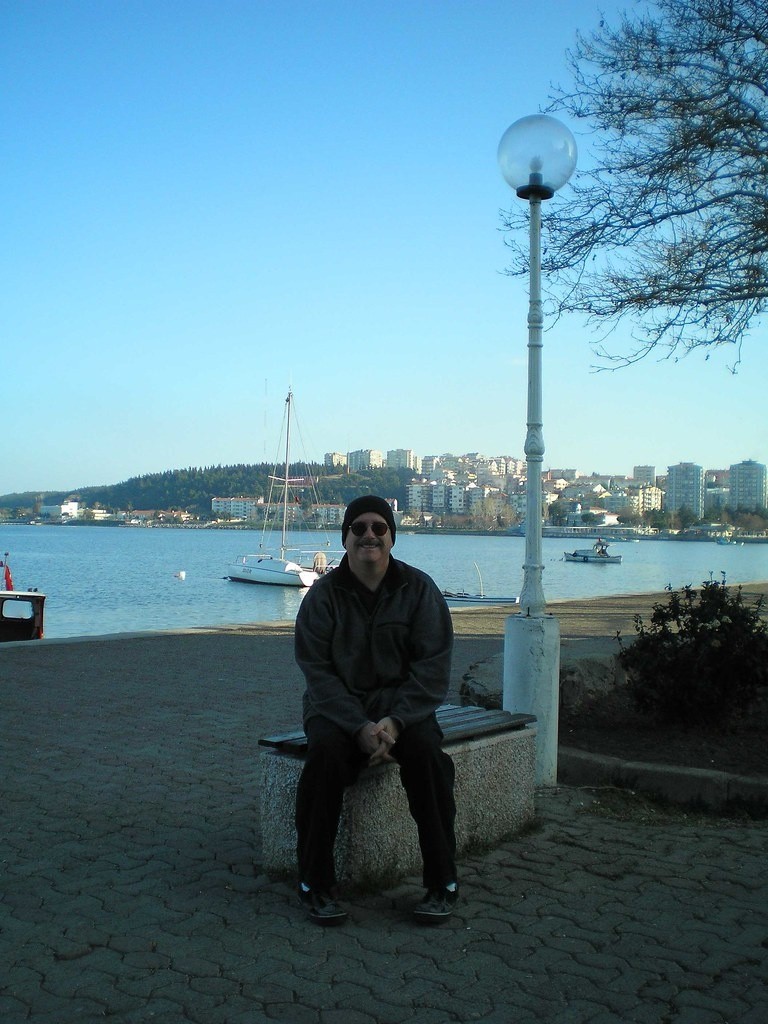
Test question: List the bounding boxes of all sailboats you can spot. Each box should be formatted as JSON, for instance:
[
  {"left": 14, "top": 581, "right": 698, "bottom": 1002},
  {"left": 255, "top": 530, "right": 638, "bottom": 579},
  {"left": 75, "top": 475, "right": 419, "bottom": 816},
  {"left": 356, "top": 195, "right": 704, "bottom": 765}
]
[{"left": 226, "top": 390, "right": 346, "bottom": 587}]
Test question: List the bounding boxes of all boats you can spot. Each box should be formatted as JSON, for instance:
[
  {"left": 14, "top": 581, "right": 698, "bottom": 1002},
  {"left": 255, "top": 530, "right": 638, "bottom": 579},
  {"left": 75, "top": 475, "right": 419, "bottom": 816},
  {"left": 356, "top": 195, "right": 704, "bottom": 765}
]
[
  {"left": 563, "top": 539, "right": 622, "bottom": 564},
  {"left": 442, "top": 560, "right": 520, "bottom": 607}
]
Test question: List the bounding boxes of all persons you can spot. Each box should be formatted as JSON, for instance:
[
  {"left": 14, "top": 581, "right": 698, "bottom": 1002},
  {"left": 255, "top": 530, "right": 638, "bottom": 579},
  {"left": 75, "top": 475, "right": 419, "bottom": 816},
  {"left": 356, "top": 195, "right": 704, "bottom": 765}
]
[{"left": 294, "top": 494, "right": 459, "bottom": 926}]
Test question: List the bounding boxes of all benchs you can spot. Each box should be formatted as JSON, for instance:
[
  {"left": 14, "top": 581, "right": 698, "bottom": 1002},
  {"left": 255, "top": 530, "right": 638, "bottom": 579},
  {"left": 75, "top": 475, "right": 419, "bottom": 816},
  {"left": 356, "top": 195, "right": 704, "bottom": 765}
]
[{"left": 255, "top": 706, "right": 540, "bottom": 885}]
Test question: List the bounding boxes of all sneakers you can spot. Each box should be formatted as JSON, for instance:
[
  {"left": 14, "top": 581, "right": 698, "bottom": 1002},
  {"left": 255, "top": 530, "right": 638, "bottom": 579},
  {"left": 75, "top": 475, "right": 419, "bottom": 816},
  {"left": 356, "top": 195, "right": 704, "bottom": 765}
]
[
  {"left": 414, "top": 881, "right": 459, "bottom": 919},
  {"left": 296, "top": 881, "right": 348, "bottom": 919}
]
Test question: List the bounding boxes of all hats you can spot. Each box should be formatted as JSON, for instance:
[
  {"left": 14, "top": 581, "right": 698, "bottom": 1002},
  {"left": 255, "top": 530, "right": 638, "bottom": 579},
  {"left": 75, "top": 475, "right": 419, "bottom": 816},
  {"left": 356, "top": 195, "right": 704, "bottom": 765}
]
[{"left": 341, "top": 495, "right": 396, "bottom": 544}]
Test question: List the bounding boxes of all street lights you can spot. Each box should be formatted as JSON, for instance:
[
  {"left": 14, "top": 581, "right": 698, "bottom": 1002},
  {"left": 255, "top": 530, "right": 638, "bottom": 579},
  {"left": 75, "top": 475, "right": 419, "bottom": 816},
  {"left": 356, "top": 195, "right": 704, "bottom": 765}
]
[{"left": 496, "top": 114, "right": 574, "bottom": 787}]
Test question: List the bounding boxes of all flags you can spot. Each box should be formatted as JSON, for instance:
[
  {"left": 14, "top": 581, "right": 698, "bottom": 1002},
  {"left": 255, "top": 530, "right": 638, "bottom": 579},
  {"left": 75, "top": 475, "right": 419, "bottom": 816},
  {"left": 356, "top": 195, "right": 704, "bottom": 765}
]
[
  {"left": 295, "top": 496, "right": 301, "bottom": 504},
  {"left": 5, "top": 566, "right": 13, "bottom": 590}
]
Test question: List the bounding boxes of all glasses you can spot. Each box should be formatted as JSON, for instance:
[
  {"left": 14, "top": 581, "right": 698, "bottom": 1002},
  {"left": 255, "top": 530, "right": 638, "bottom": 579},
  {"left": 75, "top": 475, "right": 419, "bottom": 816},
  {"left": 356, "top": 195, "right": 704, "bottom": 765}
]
[{"left": 349, "top": 522, "right": 392, "bottom": 535}]
